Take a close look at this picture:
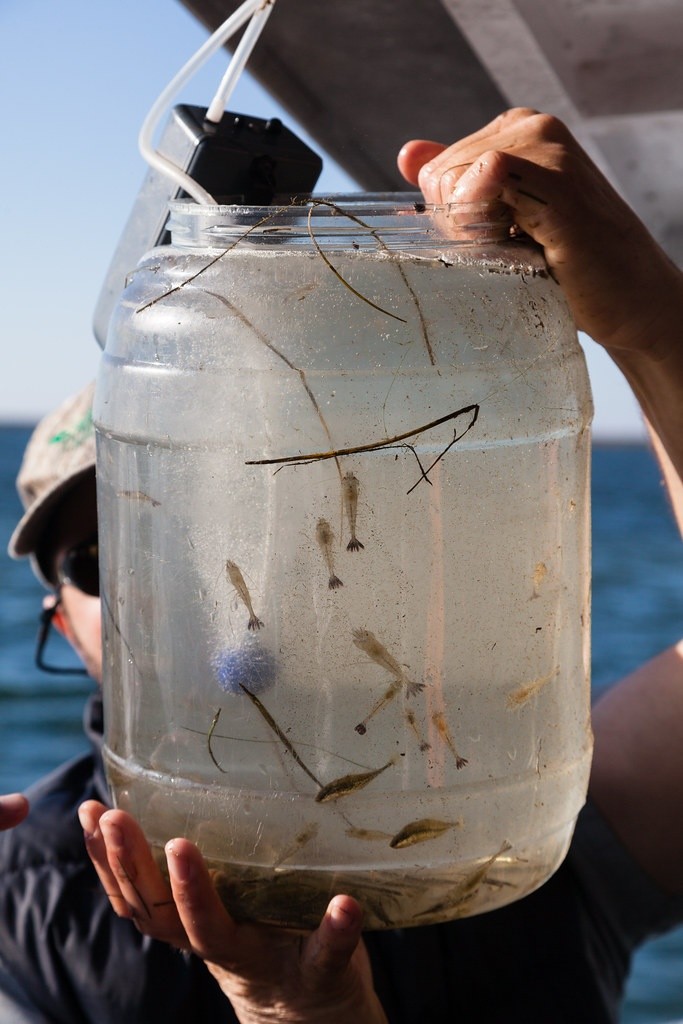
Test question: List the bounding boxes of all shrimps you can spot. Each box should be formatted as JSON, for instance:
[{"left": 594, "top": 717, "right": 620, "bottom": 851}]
[
  {"left": 351, "top": 679, "right": 404, "bottom": 736},
  {"left": 526, "top": 561, "right": 547, "bottom": 602},
  {"left": 125, "top": 820, "right": 515, "bottom": 928},
  {"left": 116, "top": 489, "right": 161, "bottom": 508},
  {"left": 431, "top": 709, "right": 469, "bottom": 770},
  {"left": 402, "top": 706, "right": 433, "bottom": 752},
  {"left": 316, "top": 518, "right": 344, "bottom": 589},
  {"left": 223, "top": 558, "right": 266, "bottom": 632},
  {"left": 341, "top": 470, "right": 366, "bottom": 553},
  {"left": 346, "top": 623, "right": 427, "bottom": 699},
  {"left": 501, "top": 664, "right": 562, "bottom": 713}
]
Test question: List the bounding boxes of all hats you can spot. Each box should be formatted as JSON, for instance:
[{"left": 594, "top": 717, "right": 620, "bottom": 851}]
[{"left": 8, "top": 377, "right": 97, "bottom": 558}]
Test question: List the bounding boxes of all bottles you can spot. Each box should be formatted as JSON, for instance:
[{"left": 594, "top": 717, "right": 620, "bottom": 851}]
[{"left": 90, "top": 194, "right": 598, "bottom": 933}]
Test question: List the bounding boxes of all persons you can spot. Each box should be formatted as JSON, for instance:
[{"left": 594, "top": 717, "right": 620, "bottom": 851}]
[
  {"left": 0, "top": 792, "right": 26, "bottom": 832},
  {"left": 0, "top": 107, "right": 683, "bottom": 1022}
]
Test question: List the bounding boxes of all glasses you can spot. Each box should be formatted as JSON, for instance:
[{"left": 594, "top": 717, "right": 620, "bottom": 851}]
[{"left": 52, "top": 536, "right": 100, "bottom": 608}]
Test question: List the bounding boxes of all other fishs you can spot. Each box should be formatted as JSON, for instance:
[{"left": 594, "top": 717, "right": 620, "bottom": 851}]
[
  {"left": 314, "top": 760, "right": 396, "bottom": 803},
  {"left": 389, "top": 819, "right": 465, "bottom": 849}
]
